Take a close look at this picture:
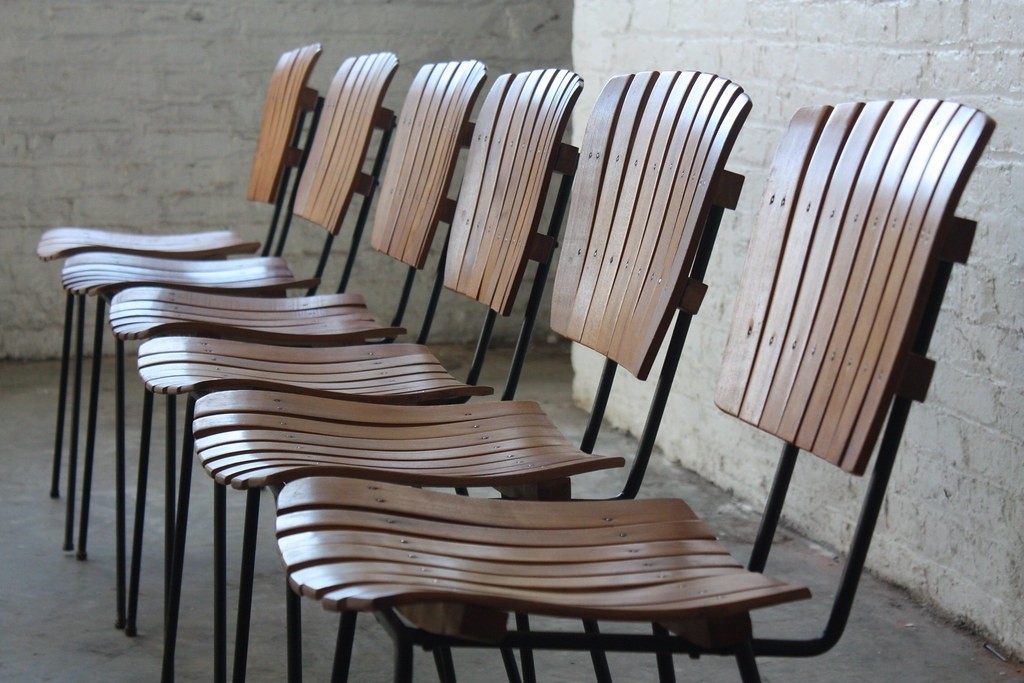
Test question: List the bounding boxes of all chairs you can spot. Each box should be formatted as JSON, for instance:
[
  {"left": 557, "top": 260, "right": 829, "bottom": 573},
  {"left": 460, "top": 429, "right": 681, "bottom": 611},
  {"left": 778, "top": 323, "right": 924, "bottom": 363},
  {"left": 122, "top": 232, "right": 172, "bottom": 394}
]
[{"left": 36, "top": 44, "right": 996, "bottom": 683}]
